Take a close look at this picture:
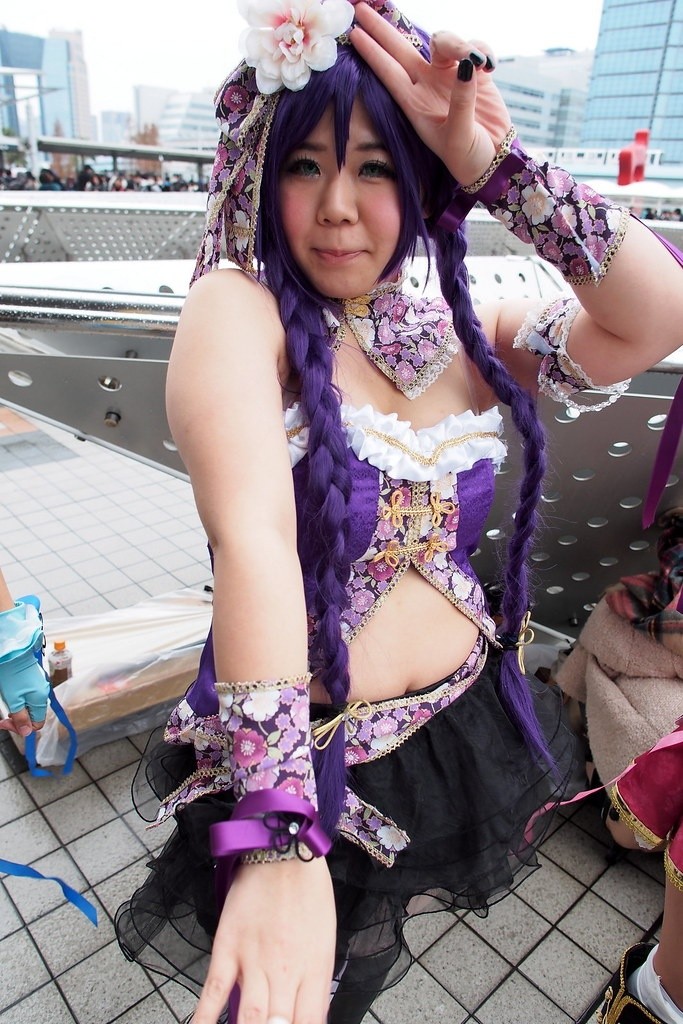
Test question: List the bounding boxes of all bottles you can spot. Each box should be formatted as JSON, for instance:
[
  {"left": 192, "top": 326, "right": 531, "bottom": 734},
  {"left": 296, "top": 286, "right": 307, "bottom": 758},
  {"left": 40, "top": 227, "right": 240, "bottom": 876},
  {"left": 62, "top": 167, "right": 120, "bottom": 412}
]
[{"left": 47, "top": 639, "right": 73, "bottom": 689}]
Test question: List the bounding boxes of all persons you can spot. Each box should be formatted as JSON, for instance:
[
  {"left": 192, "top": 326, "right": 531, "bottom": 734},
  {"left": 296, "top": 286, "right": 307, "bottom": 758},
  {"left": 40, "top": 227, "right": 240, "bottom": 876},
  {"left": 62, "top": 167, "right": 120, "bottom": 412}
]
[
  {"left": 0, "top": 165, "right": 683, "bottom": 220},
  {"left": 112, "top": 1, "right": 683, "bottom": 1024},
  {"left": 522, "top": 711, "right": 683, "bottom": 1024},
  {"left": 0, "top": 569, "right": 50, "bottom": 736}
]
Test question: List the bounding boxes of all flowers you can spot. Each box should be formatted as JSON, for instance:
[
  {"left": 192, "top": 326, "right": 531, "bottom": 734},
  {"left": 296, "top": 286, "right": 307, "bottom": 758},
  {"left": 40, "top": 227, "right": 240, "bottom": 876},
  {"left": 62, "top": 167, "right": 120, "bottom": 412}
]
[{"left": 237, "top": 0, "right": 356, "bottom": 95}]
